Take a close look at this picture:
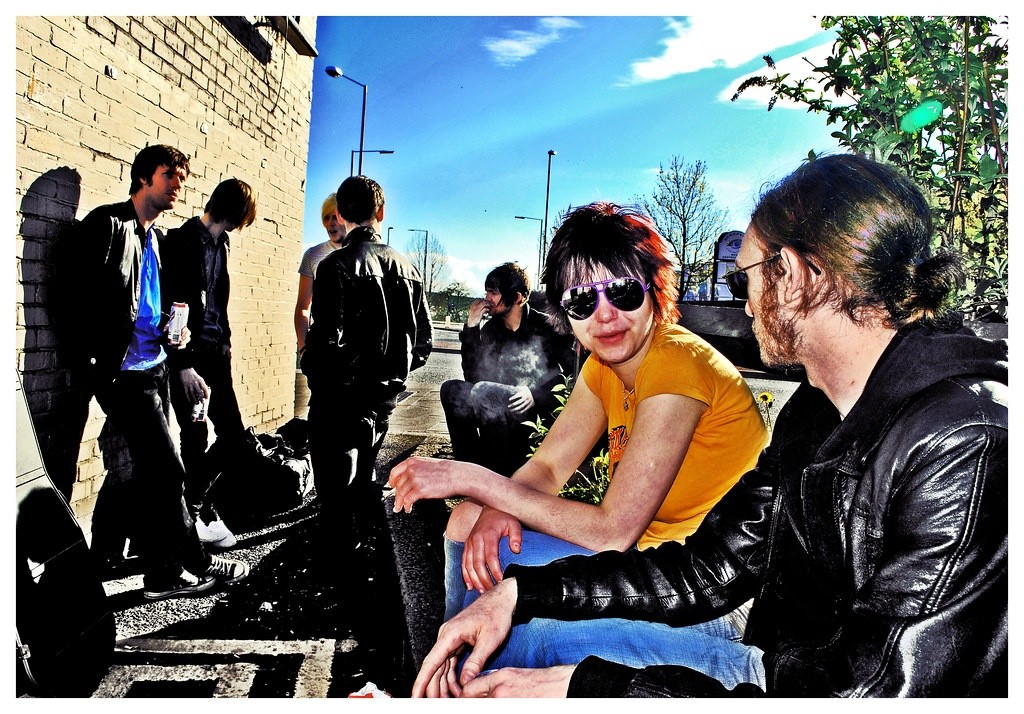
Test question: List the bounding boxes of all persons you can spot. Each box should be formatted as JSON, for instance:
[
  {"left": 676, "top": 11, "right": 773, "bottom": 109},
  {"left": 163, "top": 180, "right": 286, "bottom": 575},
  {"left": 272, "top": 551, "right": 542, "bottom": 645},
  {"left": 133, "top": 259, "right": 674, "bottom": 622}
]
[
  {"left": 294, "top": 174, "right": 432, "bottom": 534},
  {"left": 411, "top": 142, "right": 1008, "bottom": 699},
  {"left": 390, "top": 199, "right": 767, "bottom": 694},
  {"left": 440, "top": 260, "right": 588, "bottom": 478},
  {"left": 52, "top": 144, "right": 250, "bottom": 599},
  {"left": 161, "top": 176, "right": 257, "bottom": 522}
]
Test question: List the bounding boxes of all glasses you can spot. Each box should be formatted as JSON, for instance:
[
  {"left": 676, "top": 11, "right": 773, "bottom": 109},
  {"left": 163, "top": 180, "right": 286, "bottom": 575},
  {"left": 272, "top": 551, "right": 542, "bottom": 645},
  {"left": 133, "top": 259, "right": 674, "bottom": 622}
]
[
  {"left": 723, "top": 248, "right": 823, "bottom": 300},
  {"left": 560, "top": 278, "right": 650, "bottom": 321}
]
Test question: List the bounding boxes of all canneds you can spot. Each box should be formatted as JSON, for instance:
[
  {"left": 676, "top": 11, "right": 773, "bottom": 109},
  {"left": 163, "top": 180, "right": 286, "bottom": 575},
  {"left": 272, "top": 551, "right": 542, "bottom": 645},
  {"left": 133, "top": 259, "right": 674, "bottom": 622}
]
[
  {"left": 168, "top": 301, "right": 188, "bottom": 344},
  {"left": 191, "top": 385, "right": 211, "bottom": 422}
]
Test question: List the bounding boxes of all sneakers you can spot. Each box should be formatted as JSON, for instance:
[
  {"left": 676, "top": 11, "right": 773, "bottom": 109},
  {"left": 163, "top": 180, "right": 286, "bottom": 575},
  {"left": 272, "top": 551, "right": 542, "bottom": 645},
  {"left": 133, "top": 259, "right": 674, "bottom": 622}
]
[
  {"left": 143, "top": 566, "right": 215, "bottom": 601},
  {"left": 197, "top": 555, "right": 250, "bottom": 585}
]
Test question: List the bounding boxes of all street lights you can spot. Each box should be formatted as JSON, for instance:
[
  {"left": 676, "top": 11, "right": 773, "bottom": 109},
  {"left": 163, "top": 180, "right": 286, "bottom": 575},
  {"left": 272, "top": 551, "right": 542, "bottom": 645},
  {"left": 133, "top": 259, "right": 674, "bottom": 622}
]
[
  {"left": 408, "top": 228, "right": 430, "bottom": 292},
  {"left": 386, "top": 227, "right": 393, "bottom": 245},
  {"left": 515, "top": 216, "right": 543, "bottom": 293},
  {"left": 350, "top": 149, "right": 395, "bottom": 178},
  {"left": 323, "top": 65, "right": 367, "bottom": 176},
  {"left": 544, "top": 150, "right": 557, "bottom": 268}
]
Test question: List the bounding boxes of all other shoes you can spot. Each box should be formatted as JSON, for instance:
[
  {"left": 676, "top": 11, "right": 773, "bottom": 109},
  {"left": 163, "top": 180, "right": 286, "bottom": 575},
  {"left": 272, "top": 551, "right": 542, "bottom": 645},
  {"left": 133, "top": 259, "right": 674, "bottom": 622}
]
[{"left": 192, "top": 509, "right": 236, "bottom": 547}]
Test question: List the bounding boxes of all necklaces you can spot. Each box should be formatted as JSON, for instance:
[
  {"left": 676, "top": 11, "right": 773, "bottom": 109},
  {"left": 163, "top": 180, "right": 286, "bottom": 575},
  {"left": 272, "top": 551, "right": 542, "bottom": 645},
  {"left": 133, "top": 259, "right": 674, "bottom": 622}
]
[{"left": 621, "top": 381, "right": 635, "bottom": 411}]
